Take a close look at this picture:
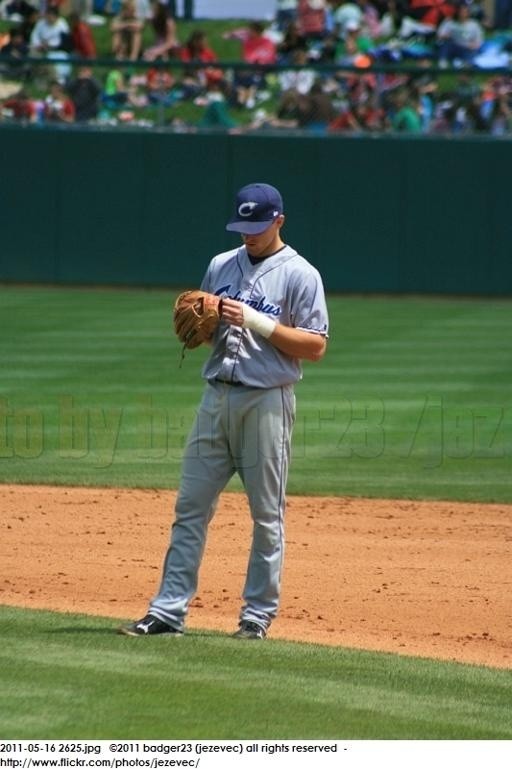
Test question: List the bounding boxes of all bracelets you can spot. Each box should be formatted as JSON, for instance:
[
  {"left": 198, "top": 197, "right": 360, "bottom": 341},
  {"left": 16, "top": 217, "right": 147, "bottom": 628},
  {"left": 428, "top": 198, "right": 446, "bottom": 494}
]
[{"left": 240, "top": 303, "right": 279, "bottom": 340}]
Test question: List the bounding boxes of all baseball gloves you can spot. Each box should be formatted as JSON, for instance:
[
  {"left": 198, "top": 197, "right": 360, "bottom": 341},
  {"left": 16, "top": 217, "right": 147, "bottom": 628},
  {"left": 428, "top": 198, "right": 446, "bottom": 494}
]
[{"left": 173, "top": 291, "right": 223, "bottom": 349}]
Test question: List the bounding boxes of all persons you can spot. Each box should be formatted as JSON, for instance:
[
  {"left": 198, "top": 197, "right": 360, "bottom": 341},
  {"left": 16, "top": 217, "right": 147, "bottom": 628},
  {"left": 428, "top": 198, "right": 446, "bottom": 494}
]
[
  {"left": 1, "top": 0, "right": 510, "bottom": 135},
  {"left": 118, "top": 180, "right": 329, "bottom": 641}
]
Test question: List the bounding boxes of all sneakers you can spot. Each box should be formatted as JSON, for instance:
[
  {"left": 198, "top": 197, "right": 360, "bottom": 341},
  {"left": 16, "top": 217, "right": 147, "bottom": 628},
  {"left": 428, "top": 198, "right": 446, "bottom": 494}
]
[
  {"left": 231, "top": 620, "right": 266, "bottom": 640},
  {"left": 120, "top": 613, "right": 184, "bottom": 638}
]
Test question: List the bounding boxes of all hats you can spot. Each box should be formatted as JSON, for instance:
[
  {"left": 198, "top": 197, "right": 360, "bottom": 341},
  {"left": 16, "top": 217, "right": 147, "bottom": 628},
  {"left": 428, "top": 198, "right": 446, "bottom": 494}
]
[{"left": 225, "top": 182, "right": 283, "bottom": 236}]
